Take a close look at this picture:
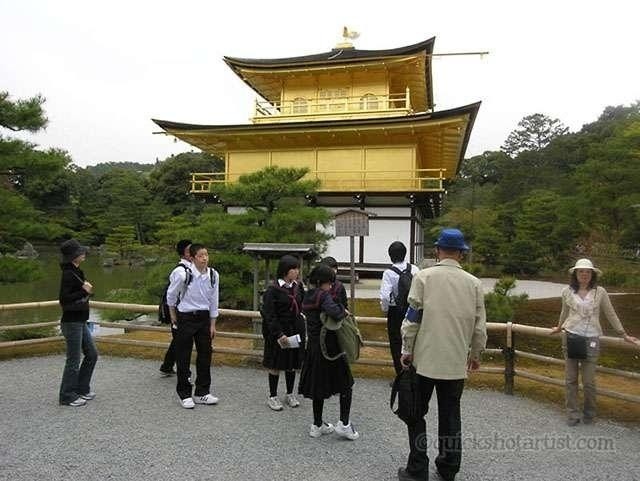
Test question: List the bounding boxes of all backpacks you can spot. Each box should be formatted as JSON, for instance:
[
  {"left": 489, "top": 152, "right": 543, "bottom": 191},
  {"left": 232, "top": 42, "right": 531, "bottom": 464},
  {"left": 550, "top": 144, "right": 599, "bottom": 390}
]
[
  {"left": 388, "top": 262, "right": 413, "bottom": 312},
  {"left": 320, "top": 308, "right": 364, "bottom": 362},
  {"left": 157, "top": 263, "right": 186, "bottom": 324}
]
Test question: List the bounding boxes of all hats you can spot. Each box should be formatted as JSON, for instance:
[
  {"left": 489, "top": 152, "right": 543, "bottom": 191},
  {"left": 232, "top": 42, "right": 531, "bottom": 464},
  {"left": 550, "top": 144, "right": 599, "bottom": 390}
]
[
  {"left": 568, "top": 259, "right": 603, "bottom": 277},
  {"left": 433, "top": 229, "right": 469, "bottom": 250},
  {"left": 59, "top": 239, "right": 89, "bottom": 264}
]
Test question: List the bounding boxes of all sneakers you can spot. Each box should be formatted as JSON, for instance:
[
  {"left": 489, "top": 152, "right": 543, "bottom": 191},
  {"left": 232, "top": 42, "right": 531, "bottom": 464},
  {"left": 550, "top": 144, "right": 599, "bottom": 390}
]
[
  {"left": 584, "top": 418, "right": 592, "bottom": 424},
  {"left": 67, "top": 397, "right": 86, "bottom": 407},
  {"left": 79, "top": 392, "right": 96, "bottom": 401},
  {"left": 179, "top": 398, "right": 195, "bottom": 409},
  {"left": 567, "top": 418, "right": 580, "bottom": 426},
  {"left": 265, "top": 395, "right": 283, "bottom": 411},
  {"left": 398, "top": 467, "right": 417, "bottom": 481},
  {"left": 309, "top": 423, "right": 333, "bottom": 438},
  {"left": 159, "top": 370, "right": 176, "bottom": 378},
  {"left": 334, "top": 421, "right": 359, "bottom": 440},
  {"left": 285, "top": 392, "right": 300, "bottom": 407},
  {"left": 194, "top": 394, "right": 218, "bottom": 404}
]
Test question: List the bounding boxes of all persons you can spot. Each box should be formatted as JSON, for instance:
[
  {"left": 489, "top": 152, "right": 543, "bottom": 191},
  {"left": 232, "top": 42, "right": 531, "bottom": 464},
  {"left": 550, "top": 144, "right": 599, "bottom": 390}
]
[
  {"left": 260, "top": 255, "right": 305, "bottom": 411},
  {"left": 58, "top": 240, "right": 98, "bottom": 408},
  {"left": 158, "top": 239, "right": 193, "bottom": 386},
  {"left": 320, "top": 256, "right": 348, "bottom": 307},
  {"left": 550, "top": 259, "right": 636, "bottom": 426},
  {"left": 380, "top": 240, "right": 420, "bottom": 387},
  {"left": 400, "top": 229, "right": 487, "bottom": 480},
  {"left": 166, "top": 244, "right": 219, "bottom": 409},
  {"left": 298, "top": 264, "right": 360, "bottom": 440}
]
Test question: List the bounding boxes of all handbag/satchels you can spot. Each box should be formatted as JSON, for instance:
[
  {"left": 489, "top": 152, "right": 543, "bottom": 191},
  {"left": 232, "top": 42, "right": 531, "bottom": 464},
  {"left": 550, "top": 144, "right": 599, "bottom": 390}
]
[
  {"left": 391, "top": 360, "right": 423, "bottom": 426},
  {"left": 59, "top": 272, "right": 91, "bottom": 311},
  {"left": 566, "top": 288, "right": 596, "bottom": 360}
]
